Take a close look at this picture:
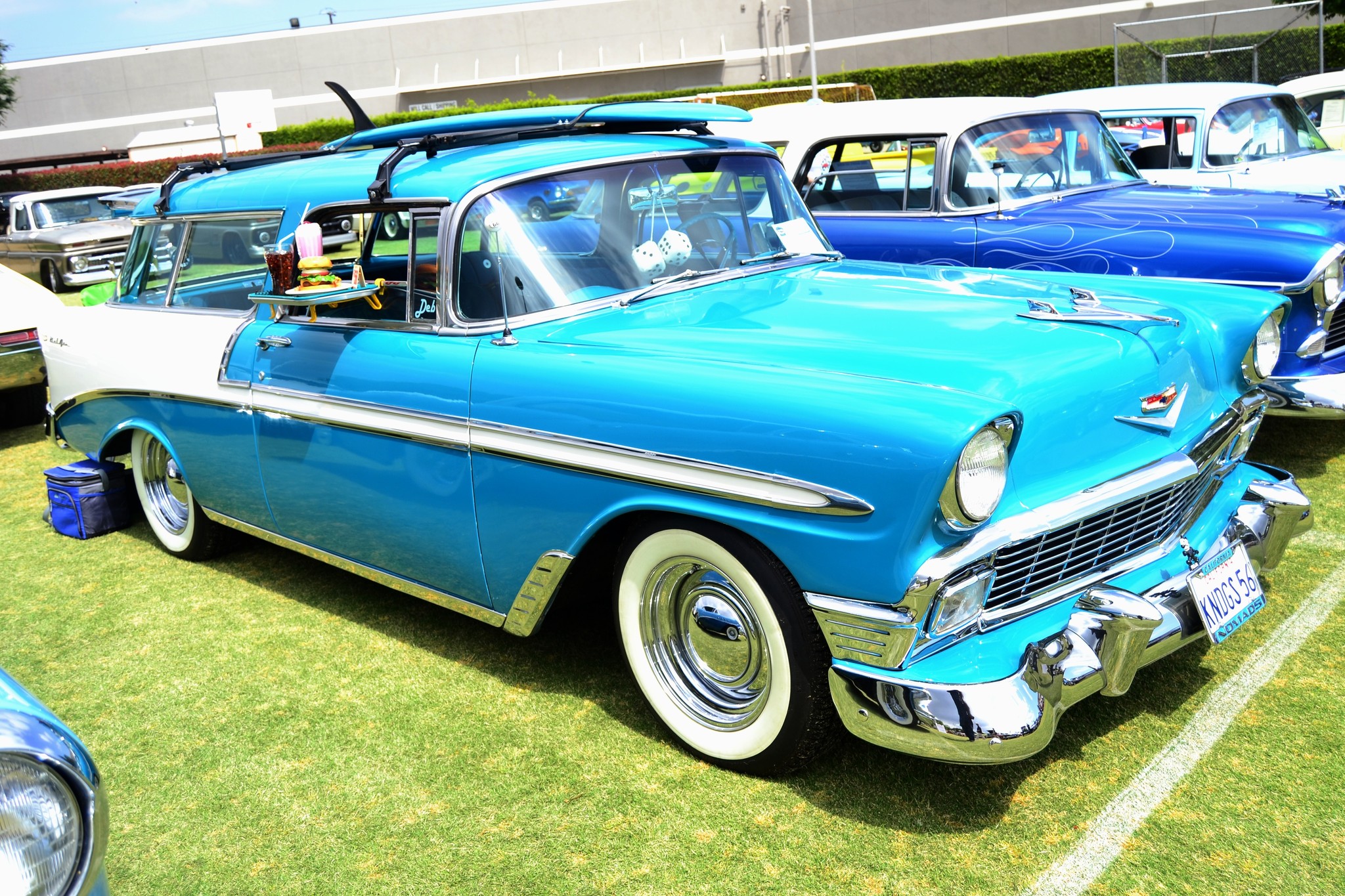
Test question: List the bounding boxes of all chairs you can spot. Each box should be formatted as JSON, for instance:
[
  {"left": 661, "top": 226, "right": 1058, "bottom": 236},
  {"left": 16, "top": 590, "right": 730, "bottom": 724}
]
[
  {"left": 454, "top": 247, "right": 567, "bottom": 318},
  {"left": 1127, "top": 143, "right": 1181, "bottom": 169}
]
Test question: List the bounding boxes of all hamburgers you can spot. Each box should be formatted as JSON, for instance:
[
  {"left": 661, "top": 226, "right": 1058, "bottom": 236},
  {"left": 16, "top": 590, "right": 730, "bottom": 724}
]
[{"left": 297, "top": 256, "right": 339, "bottom": 291}]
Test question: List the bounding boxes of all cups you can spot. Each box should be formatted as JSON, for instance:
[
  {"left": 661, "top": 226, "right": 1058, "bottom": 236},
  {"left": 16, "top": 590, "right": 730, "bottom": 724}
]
[
  {"left": 295, "top": 228, "right": 324, "bottom": 260},
  {"left": 263, "top": 243, "right": 294, "bottom": 296}
]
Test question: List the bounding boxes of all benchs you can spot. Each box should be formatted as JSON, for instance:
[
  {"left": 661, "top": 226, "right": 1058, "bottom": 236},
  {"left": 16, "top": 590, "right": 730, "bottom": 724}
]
[
  {"left": 808, "top": 193, "right": 901, "bottom": 211},
  {"left": 153, "top": 268, "right": 360, "bottom": 314}
]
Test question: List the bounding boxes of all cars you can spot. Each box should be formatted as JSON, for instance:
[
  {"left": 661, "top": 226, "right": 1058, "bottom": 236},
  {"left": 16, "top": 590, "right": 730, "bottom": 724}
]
[
  {"left": 37, "top": 75, "right": 1316, "bottom": 777},
  {"left": 0, "top": 66, "right": 1344, "bottom": 436}
]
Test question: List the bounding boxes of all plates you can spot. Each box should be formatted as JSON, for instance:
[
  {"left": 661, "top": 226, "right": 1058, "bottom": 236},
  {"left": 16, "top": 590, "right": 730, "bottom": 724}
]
[{"left": 284, "top": 283, "right": 354, "bottom": 296}]
